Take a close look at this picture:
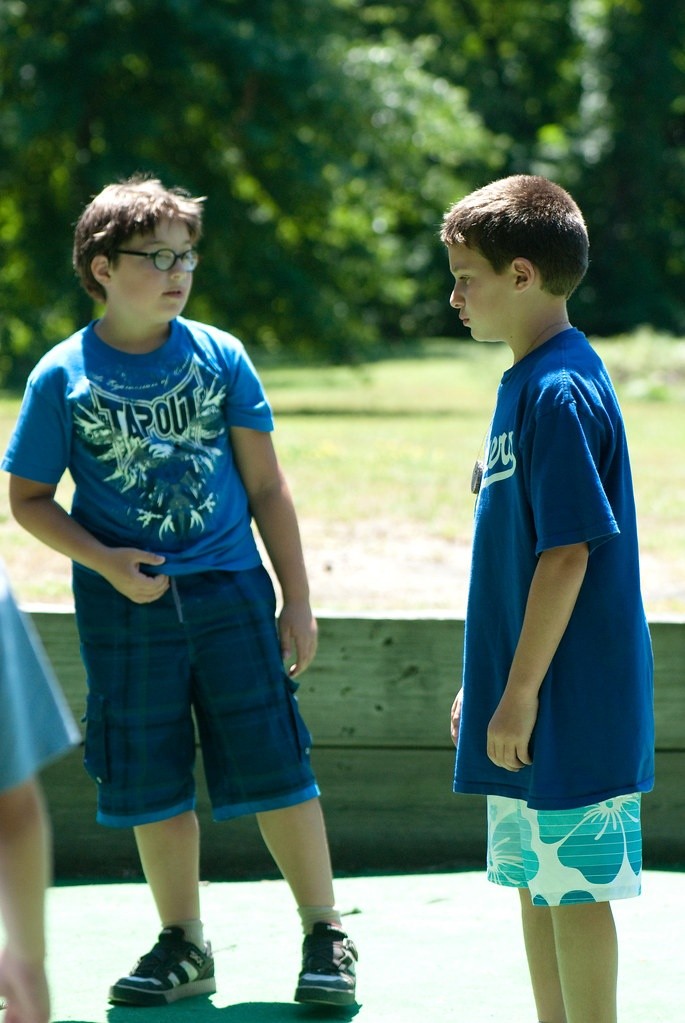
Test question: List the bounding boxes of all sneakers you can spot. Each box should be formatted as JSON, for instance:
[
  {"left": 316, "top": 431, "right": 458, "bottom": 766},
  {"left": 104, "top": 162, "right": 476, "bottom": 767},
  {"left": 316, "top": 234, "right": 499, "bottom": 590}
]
[
  {"left": 294, "top": 921, "right": 358, "bottom": 1006},
  {"left": 108, "top": 926, "right": 216, "bottom": 1005}
]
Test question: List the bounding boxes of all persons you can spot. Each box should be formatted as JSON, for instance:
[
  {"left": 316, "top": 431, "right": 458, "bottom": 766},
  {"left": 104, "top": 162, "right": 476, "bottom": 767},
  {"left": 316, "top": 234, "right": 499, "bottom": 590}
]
[
  {"left": 0, "top": 558, "right": 81, "bottom": 1023},
  {"left": 0, "top": 180, "right": 355, "bottom": 1007},
  {"left": 441, "top": 176, "right": 657, "bottom": 1023}
]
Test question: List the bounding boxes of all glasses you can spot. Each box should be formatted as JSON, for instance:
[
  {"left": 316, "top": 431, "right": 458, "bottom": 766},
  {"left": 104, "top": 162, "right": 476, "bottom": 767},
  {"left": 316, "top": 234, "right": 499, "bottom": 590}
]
[{"left": 113, "top": 249, "right": 199, "bottom": 272}]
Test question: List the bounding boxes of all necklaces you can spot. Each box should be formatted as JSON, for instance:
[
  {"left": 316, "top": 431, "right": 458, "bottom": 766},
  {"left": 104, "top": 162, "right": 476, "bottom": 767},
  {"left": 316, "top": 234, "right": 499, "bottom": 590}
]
[{"left": 471, "top": 320, "right": 572, "bottom": 495}]
